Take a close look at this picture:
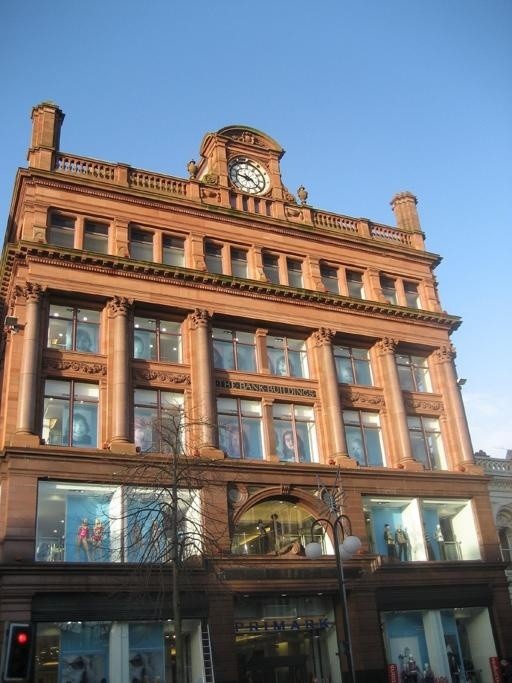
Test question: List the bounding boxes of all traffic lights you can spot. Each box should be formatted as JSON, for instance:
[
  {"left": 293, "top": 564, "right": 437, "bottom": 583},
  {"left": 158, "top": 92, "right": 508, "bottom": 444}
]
[{"left": 3, "top": 623, "right": 35, "bottom": 683}]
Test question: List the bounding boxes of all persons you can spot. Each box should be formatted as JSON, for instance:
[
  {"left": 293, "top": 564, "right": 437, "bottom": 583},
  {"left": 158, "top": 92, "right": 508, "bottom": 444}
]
[
  {"left": 406, "top": 654, "right": 434, "bottom": 683},
  {"left": 256, "top": 520, "right": 270, "bottom": 554},
  {"left": 384, "top": 523, "right": 409, "bottom": 563},
  {"left": 76, "top": 329, "right": 92, "bottom": 352},
  {"left": 224, "top": 427, "right": 251, "bottom": 458},
  {"left": 275, "top": 355, "right": 296, "bottom": 377},
  {"left": 500, "top": 657, "right": 512, "bottom": 683},
  {"left": 448, "top": 656, "right": 460, "bottom": 683},
  {"left": 131, "top": 518, "right": 161, "bottom": 562},
  {"left": 423, "top": 520, "right": 447, "bottom": 561},
  {"left": 342, "top": 367, "right": 359, "bottom": 383},
  {"left": 76, "top": 516, "right": 105, "bottom": 563},
  {"left": 280, "top": 431, "right": 307, "bottom": 463},
  {"left": 352, "top": 439, "right": 373, "bottom": 467},
  {"left": 62, "top": 414, "right": 93, "bottom": 447}
]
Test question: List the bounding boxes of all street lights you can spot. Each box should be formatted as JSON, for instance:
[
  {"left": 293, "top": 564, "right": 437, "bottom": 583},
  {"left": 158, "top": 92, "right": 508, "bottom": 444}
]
[{"left": 306, "top": 515, "right": 362, "bottom": 683}]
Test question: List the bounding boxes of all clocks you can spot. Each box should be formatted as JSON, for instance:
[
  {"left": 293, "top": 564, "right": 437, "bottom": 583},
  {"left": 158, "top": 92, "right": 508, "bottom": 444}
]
[{"left": 227, "top": 156, "right": 270, "bottom": 198}]
